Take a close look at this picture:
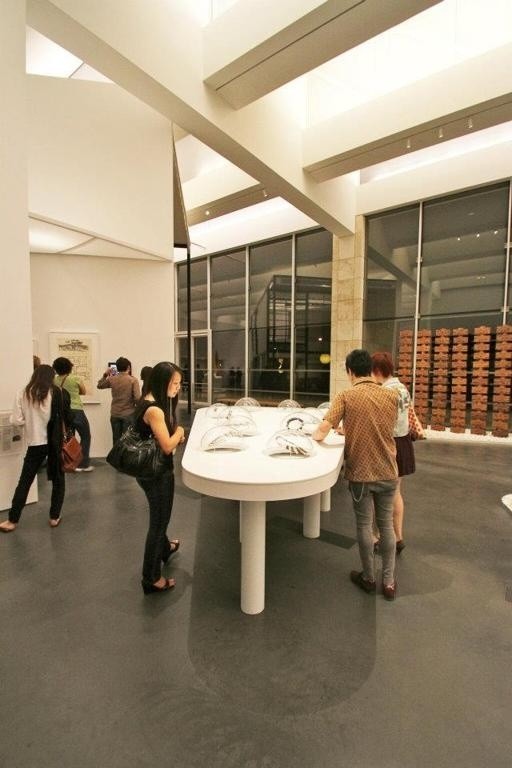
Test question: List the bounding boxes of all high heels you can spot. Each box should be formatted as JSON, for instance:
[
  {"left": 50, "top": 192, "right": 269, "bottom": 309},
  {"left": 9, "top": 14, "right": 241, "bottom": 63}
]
[
  {"left": 141, "top": 576, "right": 175, "bottom": 594},
  {"left": 396, "top": 540, "right": 405, "bottom": 554},
  {"left": 162, "top": 540, "right": 180, "bottom": 563}
]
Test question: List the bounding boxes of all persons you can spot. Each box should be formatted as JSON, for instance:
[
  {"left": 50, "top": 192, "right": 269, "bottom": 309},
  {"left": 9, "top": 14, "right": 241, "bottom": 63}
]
[
  {"left": 132, "top": 361, "right": 186, "bottom": 597},
  {"left": 235, "top": 366, "right": 243, "bottom": 394},
  {"left": 180, "top": 362, "right": 191, "bottom": 392},
  {"left": 227, "top": 365, "right": 236, "bottom": 394},
  {"left": 141, "top": 366, "right": 153, "bottom": 394},
  {"left": 333, "top": 350, "right": 416, "bottom": 555},
  {"left": 311, "top": 347, "right": 401, "bottom": 600},
  {"left": 52, "top": 354, "right": 96, "bottom": 473},
  {"left": 33, "top": 355, "right": 47, "bottom": 471},
  {"left": 0, "top": 364, "right": 71, "bottom": 534},
  {"left": 195, "top": 364, "right": 205, "bottom": 399},
  {"left": 96, "top": 357, "right": 141, "bottom": 446}
]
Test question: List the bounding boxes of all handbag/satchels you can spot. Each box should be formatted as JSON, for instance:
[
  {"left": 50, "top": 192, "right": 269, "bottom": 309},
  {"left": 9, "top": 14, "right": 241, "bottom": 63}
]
[
  {"left": 105, "top": 425, "right": 164, "bottom": 479},
  {"left": 62, "top": 435, "right": 84, "bottom": 471},
  {"left": 409, "top": 399, "right": 427, "bottom": 440}
]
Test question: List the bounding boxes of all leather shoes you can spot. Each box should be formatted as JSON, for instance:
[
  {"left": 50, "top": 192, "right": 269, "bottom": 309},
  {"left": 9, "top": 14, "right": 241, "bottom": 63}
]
[
  {"left": 383, "top": 582, "right": 397, "bottom": 600},
  {"left": 350, "top": 570, "right": 377, "bottom": 593}
]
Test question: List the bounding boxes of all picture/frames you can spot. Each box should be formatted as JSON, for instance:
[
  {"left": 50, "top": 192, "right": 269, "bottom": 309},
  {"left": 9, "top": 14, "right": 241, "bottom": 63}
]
[{"left": 46, "top": 330, "right": 101, "bottom": 404}]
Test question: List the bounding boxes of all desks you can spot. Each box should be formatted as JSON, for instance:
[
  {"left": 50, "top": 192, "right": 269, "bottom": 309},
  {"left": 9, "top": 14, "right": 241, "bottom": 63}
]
[{"left": 180, "top": 402, "right": 348, "bottom": 615}]
